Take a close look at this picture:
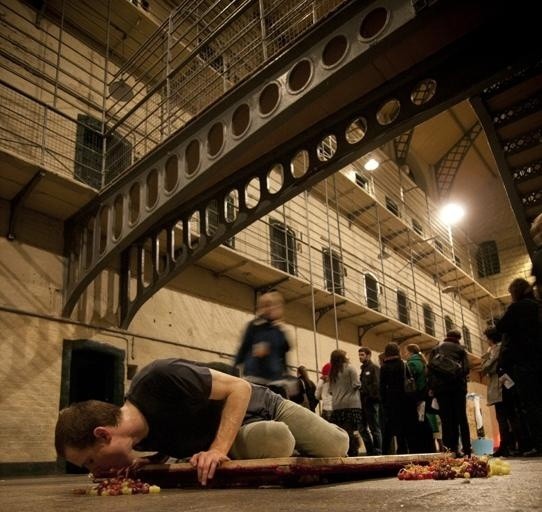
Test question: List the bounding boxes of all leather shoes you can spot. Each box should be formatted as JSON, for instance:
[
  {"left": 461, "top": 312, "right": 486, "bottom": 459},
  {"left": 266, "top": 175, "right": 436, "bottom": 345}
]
[{"left": 493, "top": 445, "right": 542, "bottom": 457}]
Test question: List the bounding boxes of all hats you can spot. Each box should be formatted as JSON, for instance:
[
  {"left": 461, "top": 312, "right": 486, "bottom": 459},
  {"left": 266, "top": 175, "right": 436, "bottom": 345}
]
[{"left": 322, "top": 363, "right": 330, "bottom": 376}]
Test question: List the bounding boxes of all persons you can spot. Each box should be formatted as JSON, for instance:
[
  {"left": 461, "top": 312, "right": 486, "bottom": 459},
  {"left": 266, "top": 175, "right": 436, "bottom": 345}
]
[
  {"left": 428, "top": 330, "right": 471, "bottom": 457},
  {"left": 232, "top": 291, "right": 291, "bottom": 401},
  {"left": 481, "top": 278, "right": 541, "bottom": 458},
  {"left": 54, "top": 357, "right": 350, "bottom": 486},
  {"left": 290, "top": 343, "right": 442, "bottom": 457}
]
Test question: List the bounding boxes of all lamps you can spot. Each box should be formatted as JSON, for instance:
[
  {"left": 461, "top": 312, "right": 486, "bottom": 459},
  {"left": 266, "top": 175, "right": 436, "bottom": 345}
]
[{"left": 109, "top": 35, "right": 134, "bottom": 102}]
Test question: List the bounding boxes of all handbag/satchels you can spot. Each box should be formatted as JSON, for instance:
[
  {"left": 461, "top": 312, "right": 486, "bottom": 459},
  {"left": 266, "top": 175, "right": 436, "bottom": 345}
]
[
  {"left": 300, "top": 393, "right": 311, "bottom": 411},
  {"left": 405, "top": 377, "right": 416, "bottom": 393},
  {"left": 430, "top": 353, "right": 461, "bottom": 381}
]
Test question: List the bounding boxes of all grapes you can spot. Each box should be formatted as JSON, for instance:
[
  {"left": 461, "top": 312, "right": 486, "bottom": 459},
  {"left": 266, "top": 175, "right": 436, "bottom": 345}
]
[
  {"left": 431, "top": 453, "right": 511, "bottom": 479},
  {"left": 86, "top": 476, "right": 161, "bottom": 496},
  {"left": 397, "top": 462, "right": 432, "bottom": 480}
]
